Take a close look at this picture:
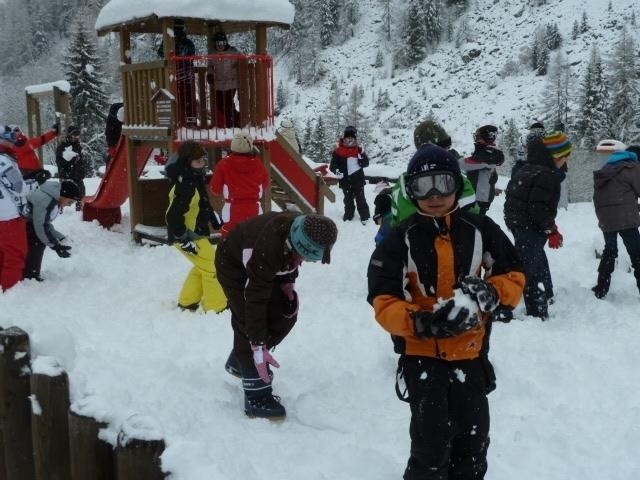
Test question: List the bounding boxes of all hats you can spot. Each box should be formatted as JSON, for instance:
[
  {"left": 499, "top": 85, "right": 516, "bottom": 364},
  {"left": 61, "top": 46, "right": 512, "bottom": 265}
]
[
  {"left": 406, "top": 121, "right": 497, "bottom": 177},
  {"left": 178, "top": 136, "right": 253, "bottom": 161},
  {"left": 291, "top": 214, "right": 337, "bottom": 264},
  {"left": 543, "top": 131, "right": 572, "bottom": 159},
  {"left": 0, "top": 124, "right": 26, "bottom": 145},
  {"left": 60, "top": 180, "right": 81, "bottom": 201},
  {"left": 344, "top": 126, "right": 357, "bottom": 138},
  {"left": 68, "top": 126, "right": 79, "bottom": 135},
  {"left": 175, "top": 20, "right": 227, "bottom": 41}
]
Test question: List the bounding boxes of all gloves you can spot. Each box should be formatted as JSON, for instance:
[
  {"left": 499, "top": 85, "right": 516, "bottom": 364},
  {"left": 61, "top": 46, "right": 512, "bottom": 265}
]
[
  {"left": 543, "top": 224, "right": 563, "bottom": 249},
  {"left": 180, "top": 238, "right": 198, "bottom": 256},
  {"left": 250, "top": 342, "right": 280, "bottom": 384},
  {"left": 452, "top": 273, "right": 500, "bottom": 310},
  {"left": 52, "top": 124, "right": 59, "bottom": 134},
  {"left": 280, "top": 282, "right": 299, "bottom": 318},
  {"left": 24, "top": 168, "right": 50, "bottom": 185},
  {"left": 207, "top": 73, "right": 215, "bottom": 84},
  {"left": 50, "top": 241, "right": 71, "bottom": 258},
  {"left": 413, "top": 300, "right": 478, "bottom": 340},
  {"left": 333, "top": 153, "right": 369, "bottom": 174}
]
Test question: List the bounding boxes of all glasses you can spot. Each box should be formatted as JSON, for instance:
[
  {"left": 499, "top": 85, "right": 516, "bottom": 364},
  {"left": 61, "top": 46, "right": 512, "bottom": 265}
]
[
  {"left": 216, "top": 40, "right": 227, "bottom": 45},
  {"left": 408, "top": 172, "right": 459, "bottom": 199}
]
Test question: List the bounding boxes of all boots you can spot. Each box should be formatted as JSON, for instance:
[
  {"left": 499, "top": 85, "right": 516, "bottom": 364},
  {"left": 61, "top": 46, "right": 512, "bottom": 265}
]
[
  {"left": 243, "top": 379, "right": 286, "bottom": 419},
  {"left": 594, "top": 275, "right": 610, "bottom": 298},
  {"left": 225, "top": 348, "right": 274, "bottom": 380}
]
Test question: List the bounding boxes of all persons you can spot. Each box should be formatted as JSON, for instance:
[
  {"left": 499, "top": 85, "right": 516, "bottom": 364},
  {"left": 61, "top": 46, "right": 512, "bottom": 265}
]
[
  {"left": 205, "top": 130, "right": 267, "bottom": 241},
  {"left": 329, "top": 126, "right": 376, "bottom": 224},
  {"left": 510, "top": 118, "right": 576, "bottom": 319},
  {"left": 157, "top": 19, "right": 198, "bottom": 128},
  {"left": 374, "top": 116, "right": 487, "bottom": 268},
  {"left": 215, "top": 211, "right": 336, "bottom": 422},
  {"left": 452, "top": 119, "right": 503, "bottom": 221},
  {"left": 159, "top": 141, "right": 232, "bottom": 315},
  {"left": 0, "top": 113, "right": 64, "bottom": 185},
  {"left": 206, "top": 31, "right": 240, "bottom": 127},
  {"left": 585, "top": 137, "right": 640, "bottom": 299},
  {"left": 53, "top": 124, "right": 91, "bottom": 209},
  {"left": 17, "top": 176, "right": 79, "bottom": 281},
  {"left": 366, "top": 142, "right": 527, "bottom": 478}
]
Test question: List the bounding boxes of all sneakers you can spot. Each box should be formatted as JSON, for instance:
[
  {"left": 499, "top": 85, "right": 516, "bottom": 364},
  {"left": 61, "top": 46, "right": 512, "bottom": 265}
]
[
  {"left": 343, "top": 214, "right": 370, "bottom": 226},
  {"left": 178, "top": 301, "right": 229, "bottom": 315},
  {"left": 22, "top": 273, "right": 44, "bottom": 281}
]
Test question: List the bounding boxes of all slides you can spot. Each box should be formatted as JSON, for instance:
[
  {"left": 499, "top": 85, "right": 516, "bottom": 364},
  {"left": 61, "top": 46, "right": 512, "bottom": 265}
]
[{"left": 81, "top": 130, "right": 153, "bottom": 230}]
